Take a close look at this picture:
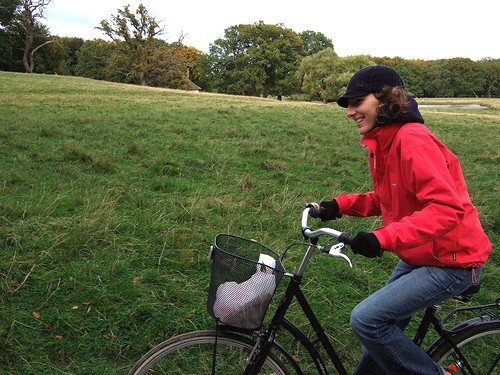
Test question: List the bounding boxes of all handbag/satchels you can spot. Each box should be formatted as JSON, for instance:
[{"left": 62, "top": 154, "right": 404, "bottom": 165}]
[{"left": 211, "top": 271, "right": 277, "bottom": 333}]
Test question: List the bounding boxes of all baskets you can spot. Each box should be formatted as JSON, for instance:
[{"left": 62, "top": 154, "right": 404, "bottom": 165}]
[{"left": 205, "top": 232, "right": 286, "bottom": 334}]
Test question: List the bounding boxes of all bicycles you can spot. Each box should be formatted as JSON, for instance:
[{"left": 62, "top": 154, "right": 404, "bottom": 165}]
[{"left": 125, "top": 200, "right": 499, "bottom": 375}]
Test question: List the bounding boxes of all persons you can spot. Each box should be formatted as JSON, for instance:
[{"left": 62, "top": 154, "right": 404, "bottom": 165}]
[{"left": 314, "top": 64, "right": 494, "bottom": 375}]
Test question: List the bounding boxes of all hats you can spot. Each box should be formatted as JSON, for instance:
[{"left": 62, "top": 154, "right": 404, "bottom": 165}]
[{"left": 336, "top": 64, "right": 407, "bottom": 108}]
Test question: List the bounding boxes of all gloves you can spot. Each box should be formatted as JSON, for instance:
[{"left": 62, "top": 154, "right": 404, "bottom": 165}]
[
  {"left": 309, "top": 198, "right": 342, "bottom": 223},
  {"left": 350, "top": 231, "right": 383, "bottom": 259}
]
